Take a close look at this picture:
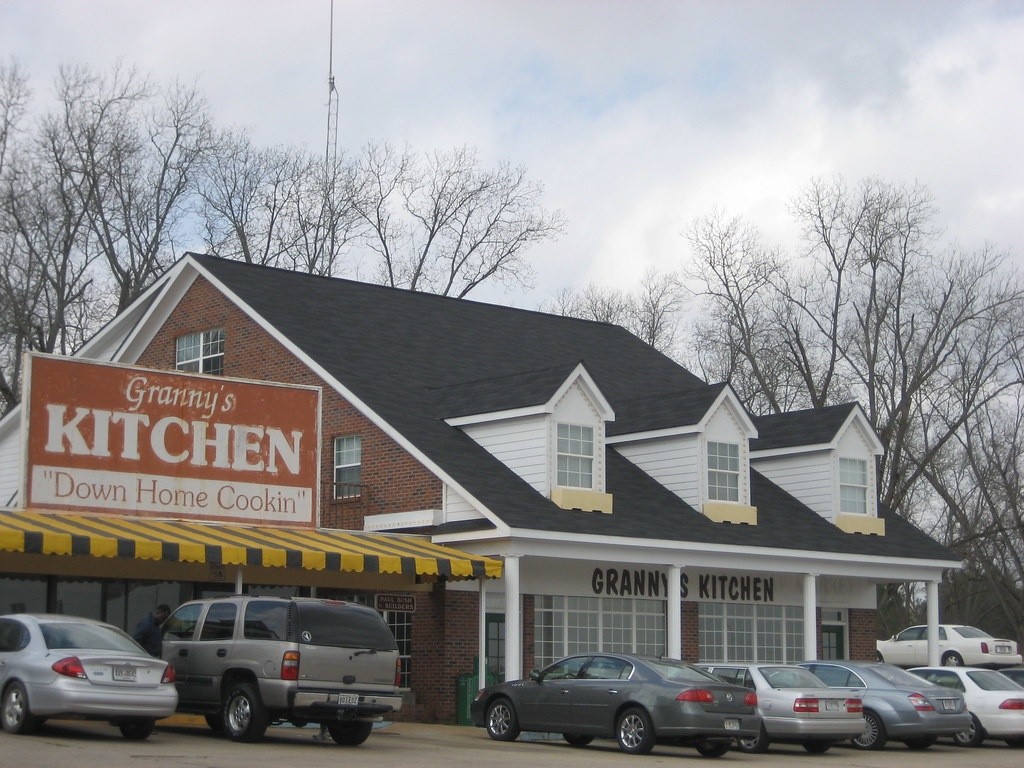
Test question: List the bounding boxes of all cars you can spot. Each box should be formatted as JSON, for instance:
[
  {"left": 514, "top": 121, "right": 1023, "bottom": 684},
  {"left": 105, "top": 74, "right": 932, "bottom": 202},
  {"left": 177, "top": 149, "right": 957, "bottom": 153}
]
[
  {"left": 470, "top": 650, "right": 763, "bottom": 758},
  {"left": 0, "top": 612, "right": 179, "bottom": 741},
  {"left": 876, "top": 624, "right": 1023, "bottom": 670},
  {"left": 770, "top": 659, "right": 973, "bottom": 752},
  {"left": 886, "top": 665, "right": 1023, "bottom": 748},
  {"left": 666, "top": 661, "right": 867, "bottom": 754}
]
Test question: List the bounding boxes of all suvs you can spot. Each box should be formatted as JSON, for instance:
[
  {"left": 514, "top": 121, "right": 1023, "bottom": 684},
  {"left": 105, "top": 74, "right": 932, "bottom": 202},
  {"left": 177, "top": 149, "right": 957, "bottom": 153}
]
[{"left": 156, "top": 593, "right": 407, "bottom": 747}]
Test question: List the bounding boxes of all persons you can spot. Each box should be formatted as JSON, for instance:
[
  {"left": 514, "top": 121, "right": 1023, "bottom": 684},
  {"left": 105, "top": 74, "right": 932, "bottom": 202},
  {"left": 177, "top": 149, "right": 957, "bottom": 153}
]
[{"left": 133, "top": 604, "right": 171, "bottom": 658}]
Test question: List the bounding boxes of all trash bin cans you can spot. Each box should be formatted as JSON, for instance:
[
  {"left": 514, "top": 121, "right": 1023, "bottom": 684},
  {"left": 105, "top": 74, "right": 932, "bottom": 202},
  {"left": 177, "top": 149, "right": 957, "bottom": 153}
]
[{"left": 456, "top": 655, "right": 500, "bottom": 727}]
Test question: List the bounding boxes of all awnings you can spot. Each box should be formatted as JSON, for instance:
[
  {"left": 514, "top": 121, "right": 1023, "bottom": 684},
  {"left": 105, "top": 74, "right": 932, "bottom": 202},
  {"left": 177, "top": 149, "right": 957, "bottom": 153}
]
[{"left": 1, "top": 510, "right": 504, "bottom": 583}]
